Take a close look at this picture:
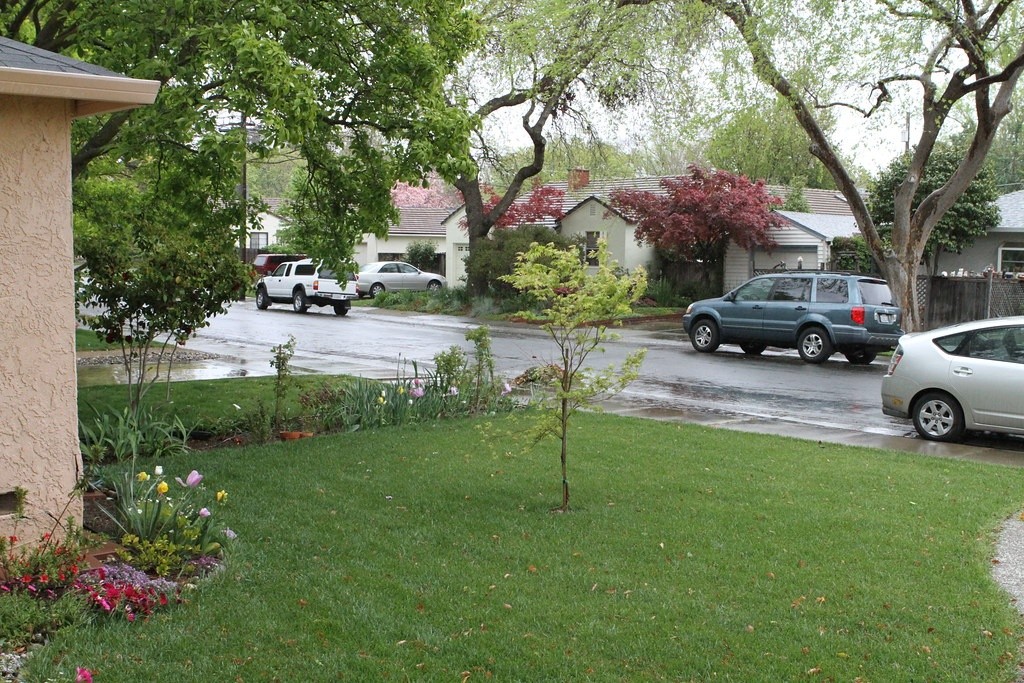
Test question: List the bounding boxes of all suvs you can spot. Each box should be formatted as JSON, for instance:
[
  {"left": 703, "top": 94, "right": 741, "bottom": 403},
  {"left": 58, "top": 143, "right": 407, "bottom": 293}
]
[
  {"left": 253, "top": 259, "right": 359, "bottom": 316},
  {"left": 681, "top": 269, "right": 905, "bottom": 366}
]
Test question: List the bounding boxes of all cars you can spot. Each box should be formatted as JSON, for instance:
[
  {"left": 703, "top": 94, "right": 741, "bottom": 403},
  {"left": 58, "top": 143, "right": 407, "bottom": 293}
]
[
  {"left": 357, "top": 259, "right": 448, "bottom": 299},
  {"left": 881, "top": 313, "right": 1024, "bottom": 442}
]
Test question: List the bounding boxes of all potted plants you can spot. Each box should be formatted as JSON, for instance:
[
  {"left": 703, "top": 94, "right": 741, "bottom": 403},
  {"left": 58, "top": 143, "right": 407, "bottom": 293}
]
[{"left": 982, "top": 265, "right": 996, "bottom": 279}]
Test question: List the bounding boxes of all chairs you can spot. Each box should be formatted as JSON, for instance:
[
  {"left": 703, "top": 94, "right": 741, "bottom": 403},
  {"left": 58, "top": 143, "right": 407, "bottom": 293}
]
[
  {"left": 777, "top": 281, "right": 793, "bottom": 300},
  {"left": 1000, "top": 331, "right": 1024, "bottom": 361}
]
